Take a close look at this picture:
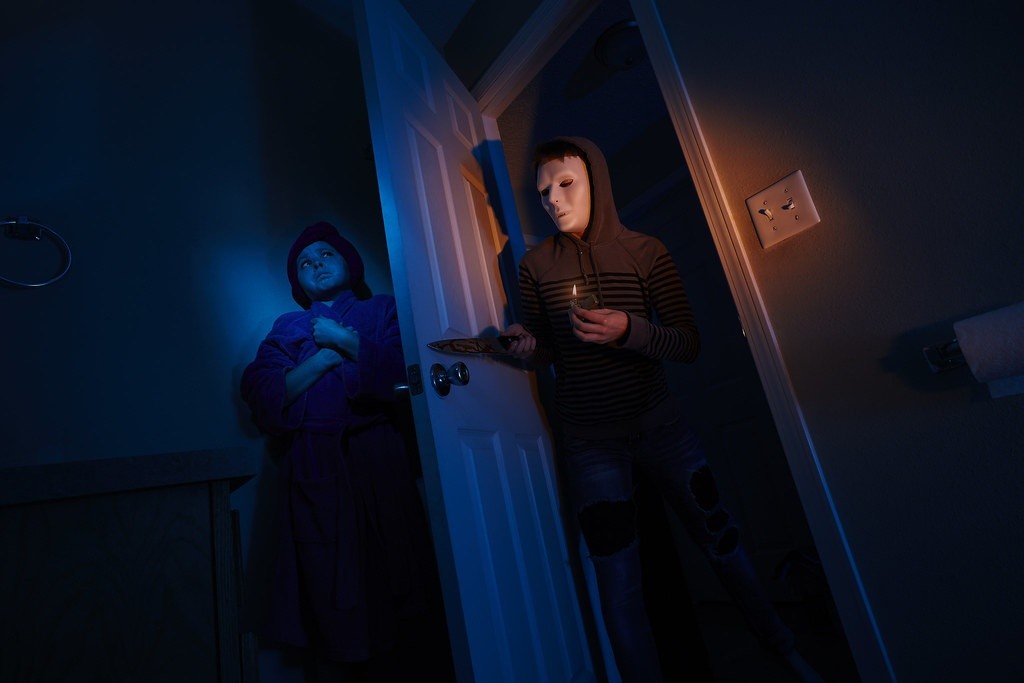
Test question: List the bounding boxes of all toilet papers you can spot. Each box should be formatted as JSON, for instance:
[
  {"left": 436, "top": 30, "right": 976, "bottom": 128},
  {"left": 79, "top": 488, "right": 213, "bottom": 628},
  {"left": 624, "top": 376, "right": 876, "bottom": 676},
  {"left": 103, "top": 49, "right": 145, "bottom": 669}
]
[{"left": 953, "top": 300, "right": 1024, "bottom": 385}]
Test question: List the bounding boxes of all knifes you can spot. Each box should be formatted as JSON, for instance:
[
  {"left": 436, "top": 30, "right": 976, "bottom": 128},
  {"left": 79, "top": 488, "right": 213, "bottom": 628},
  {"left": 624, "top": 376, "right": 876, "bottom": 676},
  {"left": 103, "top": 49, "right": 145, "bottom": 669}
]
[{"left": 427, "top": 336, "right": 523, "bottom": 354}]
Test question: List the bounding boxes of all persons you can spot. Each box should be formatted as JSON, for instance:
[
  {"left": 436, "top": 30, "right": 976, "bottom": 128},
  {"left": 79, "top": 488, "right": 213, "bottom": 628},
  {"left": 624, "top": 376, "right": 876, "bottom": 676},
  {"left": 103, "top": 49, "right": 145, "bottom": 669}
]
[
  {"left": 500, "top": 136, "right": 825, "bottom": 683},
  {"left": 238, "top": 222, "right": 458, "bottom": 682}
]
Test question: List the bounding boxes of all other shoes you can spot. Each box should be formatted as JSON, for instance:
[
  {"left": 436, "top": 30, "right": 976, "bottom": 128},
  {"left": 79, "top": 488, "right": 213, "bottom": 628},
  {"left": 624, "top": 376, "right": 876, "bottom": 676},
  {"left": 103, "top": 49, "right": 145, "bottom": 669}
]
[{"left": 781, "top": 650, "right": 823, "bottom": 683}]
